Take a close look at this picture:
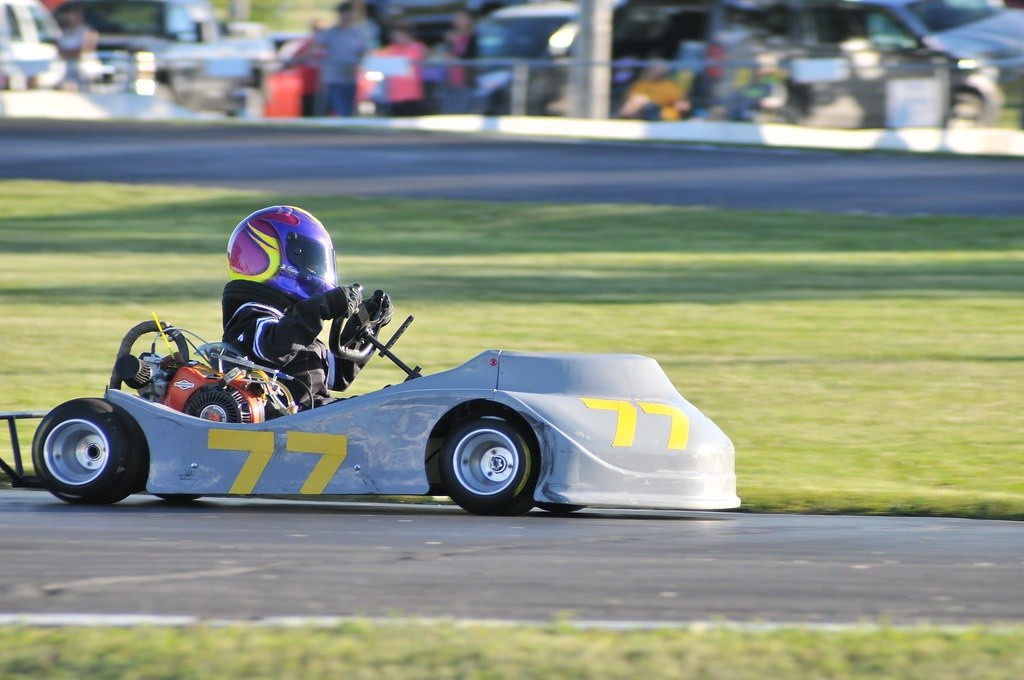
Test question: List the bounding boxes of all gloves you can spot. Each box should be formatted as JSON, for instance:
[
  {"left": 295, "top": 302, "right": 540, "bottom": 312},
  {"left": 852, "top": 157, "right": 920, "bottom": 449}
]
[
  {"left": 354, "top": 289, "right": 395, "bottom": 333},
  {"left": 314, "top": 280, "right": 362, "bottom": 320}
]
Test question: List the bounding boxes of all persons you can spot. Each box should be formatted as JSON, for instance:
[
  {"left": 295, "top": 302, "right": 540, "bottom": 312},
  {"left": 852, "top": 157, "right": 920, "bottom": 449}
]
[
  {"left": 304, "top": 2, "right": 370, "bottom": 116},
  {"left": 445, "top": 9, "right": 481, "bottom": 90},
  {"left": 223, "top": 204, "right": 393, "bottom": 412},
  {"left": 53, "top": 3, "right": 104, "bottom": 84},
  {"left": 372, "top": 26, "right": 432, "bottom": 117},
  {"left": 628, "top": 59, "right": 693, "bottom": 122}
]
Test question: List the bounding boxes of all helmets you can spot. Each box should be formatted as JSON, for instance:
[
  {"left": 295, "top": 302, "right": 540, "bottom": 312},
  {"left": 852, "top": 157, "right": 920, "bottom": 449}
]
[{"left": 226, "top": 203, "right": 337, "bottom": 301}]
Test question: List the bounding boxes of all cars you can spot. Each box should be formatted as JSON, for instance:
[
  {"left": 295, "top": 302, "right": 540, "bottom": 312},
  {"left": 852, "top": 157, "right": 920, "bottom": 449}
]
[{"left": 1, "top": 0, "right": 1024, "bottom": 131}]
